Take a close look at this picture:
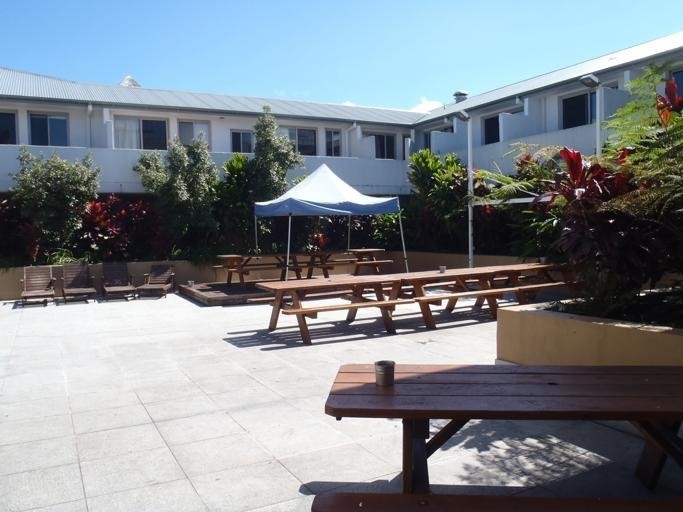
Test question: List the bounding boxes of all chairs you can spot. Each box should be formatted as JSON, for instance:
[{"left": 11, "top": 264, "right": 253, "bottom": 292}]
[{"left": 18, "top": 261, "right": 176, "bottom": 307}]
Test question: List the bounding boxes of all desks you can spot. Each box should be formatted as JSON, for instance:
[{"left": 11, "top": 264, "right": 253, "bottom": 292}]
[
  {"left": 367, "top": 263, "right": 561, "bottom": 329},
  {"left": 323, "top": 360, "right": 682, "bottom": 494},
  {"left": 215, "top": 248, "right": 385, "bottom": 288}
]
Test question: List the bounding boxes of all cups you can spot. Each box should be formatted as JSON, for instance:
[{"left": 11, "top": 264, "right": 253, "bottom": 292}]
[
  {"left": 374, "top": 361, "right": 395, "bottom": 387},
  {"left": 440, "top": 266, "right": 446, "bottom": 272}
]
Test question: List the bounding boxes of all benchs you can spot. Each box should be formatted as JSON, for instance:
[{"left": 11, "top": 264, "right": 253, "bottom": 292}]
[
  {"left": 310, "top": 491, "right": 682, "bottom": 512},
  {"left": 210, "top": 257, "right": 394, "bottom": 291},
  {"left": 246, "top": 274, "right": 577, "bottom": 346}
]
